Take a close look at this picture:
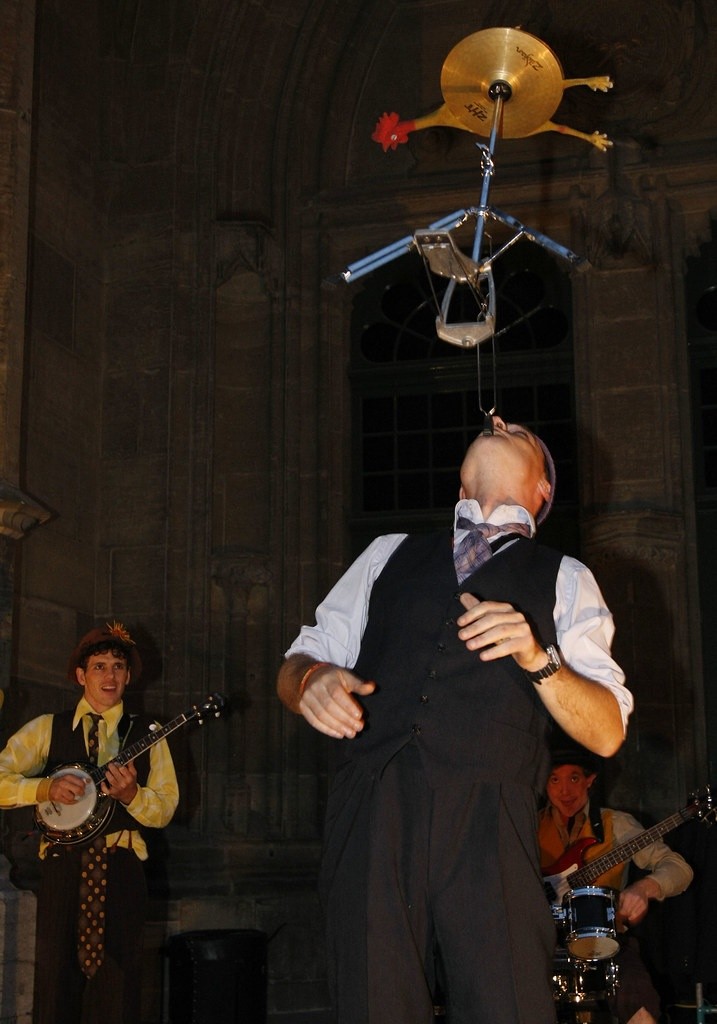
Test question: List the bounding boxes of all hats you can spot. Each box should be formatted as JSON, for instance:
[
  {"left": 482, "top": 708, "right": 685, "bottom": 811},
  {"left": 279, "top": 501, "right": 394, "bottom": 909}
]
[
  {"left": 535, "top": 434, "right": 556, "bottom": 525},
  {"left": 68, "top": 620, "right": 142, "bottom": 686},
  {"left": 550, "top": 732, "right": 597, "bottom": 778}
]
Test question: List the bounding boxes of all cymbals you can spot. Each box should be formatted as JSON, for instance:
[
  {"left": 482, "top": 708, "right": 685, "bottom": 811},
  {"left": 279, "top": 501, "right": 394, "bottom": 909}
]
[{"left": 438, "top": 26, "right": 565, "bottom": 140}]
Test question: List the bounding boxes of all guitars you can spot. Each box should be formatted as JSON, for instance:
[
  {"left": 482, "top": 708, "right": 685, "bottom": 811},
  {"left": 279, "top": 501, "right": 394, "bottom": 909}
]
[
  {"left": 31, "top": 691, "right": 228, "bottom": 849},
  {"left": 541, "top": 783, "right": 717, "bottom": 933}
]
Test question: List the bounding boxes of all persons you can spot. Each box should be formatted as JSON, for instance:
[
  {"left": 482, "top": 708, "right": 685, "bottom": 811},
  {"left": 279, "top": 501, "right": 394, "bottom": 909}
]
[
  {"left": 0, "top": 625, "right": 180, "bottom": 1024},
  {"left": 274, "top": 413, "right": 694, "bottom": 1024}
]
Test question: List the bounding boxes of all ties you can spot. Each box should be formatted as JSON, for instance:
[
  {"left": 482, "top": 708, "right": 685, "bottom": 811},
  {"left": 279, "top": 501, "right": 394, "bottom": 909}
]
[
  {"left": 78, "top": 712, "right": 107, "bottom": 979},
  {"left": 455, "top": 517, "right": 530, "bottom": 585}
]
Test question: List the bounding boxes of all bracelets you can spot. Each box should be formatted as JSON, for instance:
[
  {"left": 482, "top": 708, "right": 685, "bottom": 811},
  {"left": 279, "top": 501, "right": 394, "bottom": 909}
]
[{"left": 298, "top": 662, "right": 332, "bottom": 702}]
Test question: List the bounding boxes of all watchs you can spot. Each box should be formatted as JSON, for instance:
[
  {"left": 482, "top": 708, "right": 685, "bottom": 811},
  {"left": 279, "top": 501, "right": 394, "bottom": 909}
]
[{"left": 518, "top": 642, "right": 562, "bottom": 685}]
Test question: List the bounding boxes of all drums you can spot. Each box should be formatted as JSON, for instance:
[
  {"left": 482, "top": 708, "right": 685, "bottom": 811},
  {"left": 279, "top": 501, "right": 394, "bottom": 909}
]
[
  {"left": 545, "top": 946, "right": 622, "bottom": 1002},
  {"left": 560, "top": 885, "right": 622, "bottom": 961}
]
